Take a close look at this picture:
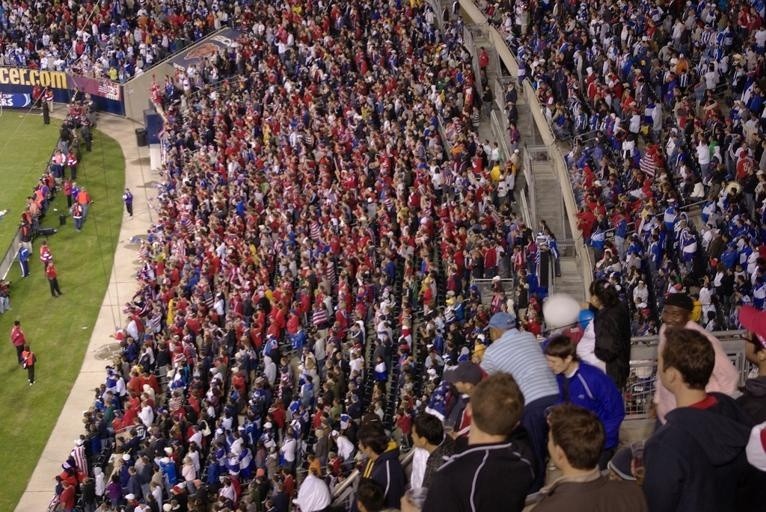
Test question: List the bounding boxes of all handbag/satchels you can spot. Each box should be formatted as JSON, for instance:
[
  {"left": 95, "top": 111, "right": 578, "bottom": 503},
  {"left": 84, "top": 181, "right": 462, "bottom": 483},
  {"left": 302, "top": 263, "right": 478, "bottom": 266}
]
[{"left": 21, "top": 362, "right": 26, "bottom": 369}]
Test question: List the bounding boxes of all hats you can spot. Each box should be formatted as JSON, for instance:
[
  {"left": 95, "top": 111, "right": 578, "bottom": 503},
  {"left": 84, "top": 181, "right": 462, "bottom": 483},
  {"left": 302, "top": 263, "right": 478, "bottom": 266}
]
[
  {"left": 482, "top": 312, "right": 517, "bottom": 332},
  {"left": 443, "top": 362, "right": 481, "bottom": 384},
  {"left": 738, "top": 304, "right": 766, "bottom": 348},
  {"left": 662, "top": 293, "right": 694, "bottom": 311}
]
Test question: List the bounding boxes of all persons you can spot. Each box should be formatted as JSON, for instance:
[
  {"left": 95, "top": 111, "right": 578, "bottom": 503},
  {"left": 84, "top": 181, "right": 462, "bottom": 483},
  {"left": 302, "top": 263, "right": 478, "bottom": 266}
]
[{"left": 0, "top": 2, "right": 765, "bottom": 511}]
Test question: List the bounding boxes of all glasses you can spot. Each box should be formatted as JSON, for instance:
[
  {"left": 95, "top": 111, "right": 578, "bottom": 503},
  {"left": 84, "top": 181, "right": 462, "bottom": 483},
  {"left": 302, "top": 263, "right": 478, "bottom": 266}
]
[{"left": 738, "top": 333, "right": 756, "bottom": 345}]
